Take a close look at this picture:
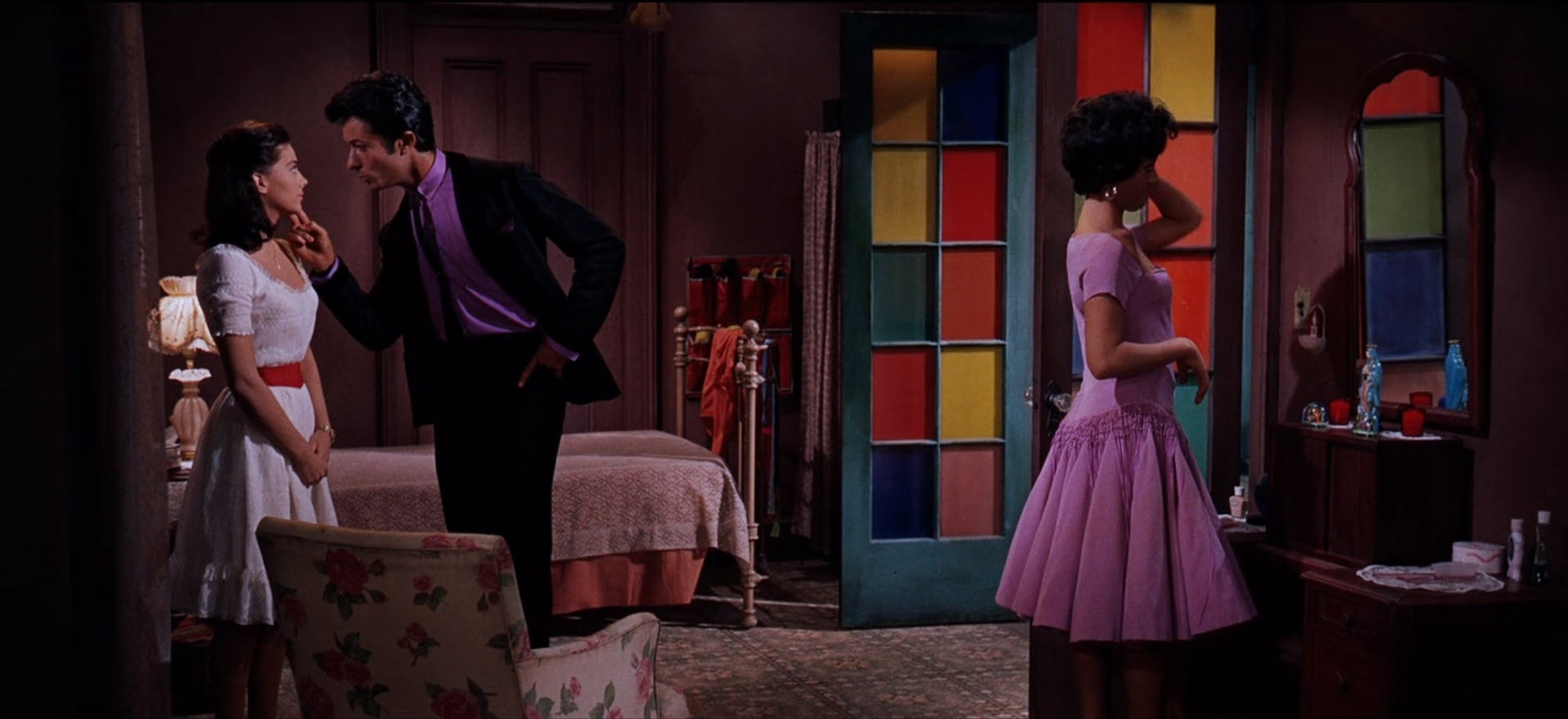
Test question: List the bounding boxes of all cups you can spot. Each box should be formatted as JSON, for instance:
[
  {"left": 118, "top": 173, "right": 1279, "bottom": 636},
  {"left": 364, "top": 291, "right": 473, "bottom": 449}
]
[
  {"left": 1410, "top": 392, "right": 1432, "bottom": 406},
  {"left": 1401, "top": 405, "right": 1427, "bottom": 437},
  {"left": 1329, "top": 395, "right": 1352, "bottom": 425}
]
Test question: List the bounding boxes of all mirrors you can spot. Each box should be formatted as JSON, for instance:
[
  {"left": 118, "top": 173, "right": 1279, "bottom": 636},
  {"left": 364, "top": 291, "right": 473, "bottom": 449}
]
[{"left": 1342, "top": 51, "right": 1492, "bottom": 440}]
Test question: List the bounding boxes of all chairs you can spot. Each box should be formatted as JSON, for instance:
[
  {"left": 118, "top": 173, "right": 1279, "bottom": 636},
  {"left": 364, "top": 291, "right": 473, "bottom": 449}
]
[{"left": 252, "top": 516, "right": 693, "bottom": 719}]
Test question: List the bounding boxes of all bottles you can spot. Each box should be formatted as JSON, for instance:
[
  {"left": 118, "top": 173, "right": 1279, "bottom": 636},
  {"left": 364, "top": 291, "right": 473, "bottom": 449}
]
[
  {"left": 1531, "top": 511, "right": 1550, "bottom": 583},
  {"left": 1229, "top": 486, "right": 1249, "bottom": 523},
  {"left": 1506, "top": 519, "right": 1526, "bottom": 582}
]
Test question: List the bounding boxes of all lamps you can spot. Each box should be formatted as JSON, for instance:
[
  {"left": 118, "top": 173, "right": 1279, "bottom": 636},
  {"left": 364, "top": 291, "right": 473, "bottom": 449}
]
[{"left": 146, "top": 274, "right": 222, "bottom": 482}]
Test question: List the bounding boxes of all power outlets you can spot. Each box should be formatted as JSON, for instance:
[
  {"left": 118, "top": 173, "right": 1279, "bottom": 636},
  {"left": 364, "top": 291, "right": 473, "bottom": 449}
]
[{"left": 1294, "top": 287, "right": 1310, "bottom": 331}]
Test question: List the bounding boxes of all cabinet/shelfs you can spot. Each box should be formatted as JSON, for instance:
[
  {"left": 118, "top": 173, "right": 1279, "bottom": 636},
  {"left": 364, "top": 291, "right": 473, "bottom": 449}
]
[{"left": 1219, "top": 417, "right": 1568, "bottom": 719}]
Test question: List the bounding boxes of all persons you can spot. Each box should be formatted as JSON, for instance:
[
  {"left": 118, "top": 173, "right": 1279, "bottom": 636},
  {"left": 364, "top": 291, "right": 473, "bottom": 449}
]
[
  {"left": 994, "top": 90, "right": 1259, "bottom": 719},
  {"left": 1357, "top": 348, "right": 1382, "bottom": 432},
  {"left": 172, "top": 119, "right": 340, "bottom": 719},
  {"left": 289, "top": 71, "right": 627, "bottom": 652},
  {"left": 1444, "top": 344, "right": 1467, "bottom": 411}
]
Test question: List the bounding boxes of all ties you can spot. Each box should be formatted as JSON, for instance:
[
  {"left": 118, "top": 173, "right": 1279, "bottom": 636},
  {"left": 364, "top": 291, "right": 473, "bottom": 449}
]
[{"left": 412, "top": 192, "right": 467, "bottom": 350}]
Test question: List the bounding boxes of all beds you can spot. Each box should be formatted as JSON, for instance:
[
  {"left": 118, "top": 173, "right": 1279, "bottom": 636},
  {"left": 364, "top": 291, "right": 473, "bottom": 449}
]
[{"left": 164, "top": 304, "right": 770, "bottom": 629}]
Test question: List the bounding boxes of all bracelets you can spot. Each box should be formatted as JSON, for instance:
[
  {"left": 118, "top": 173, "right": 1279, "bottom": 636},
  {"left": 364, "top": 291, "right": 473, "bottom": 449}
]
[{"left": 316, "top": 425, "right": 335, "bottom": 442}]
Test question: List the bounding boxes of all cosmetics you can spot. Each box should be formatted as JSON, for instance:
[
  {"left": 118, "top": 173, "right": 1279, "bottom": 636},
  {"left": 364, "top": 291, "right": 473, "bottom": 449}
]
[{"left": 1229, "top": 486, "right": 1248, "bottom": 518}]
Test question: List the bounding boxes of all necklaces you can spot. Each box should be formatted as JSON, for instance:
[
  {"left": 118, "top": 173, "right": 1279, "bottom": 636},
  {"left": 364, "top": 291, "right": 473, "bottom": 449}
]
[{"left": 265, "top": 242, "right": 281, "bottom": 270}]
